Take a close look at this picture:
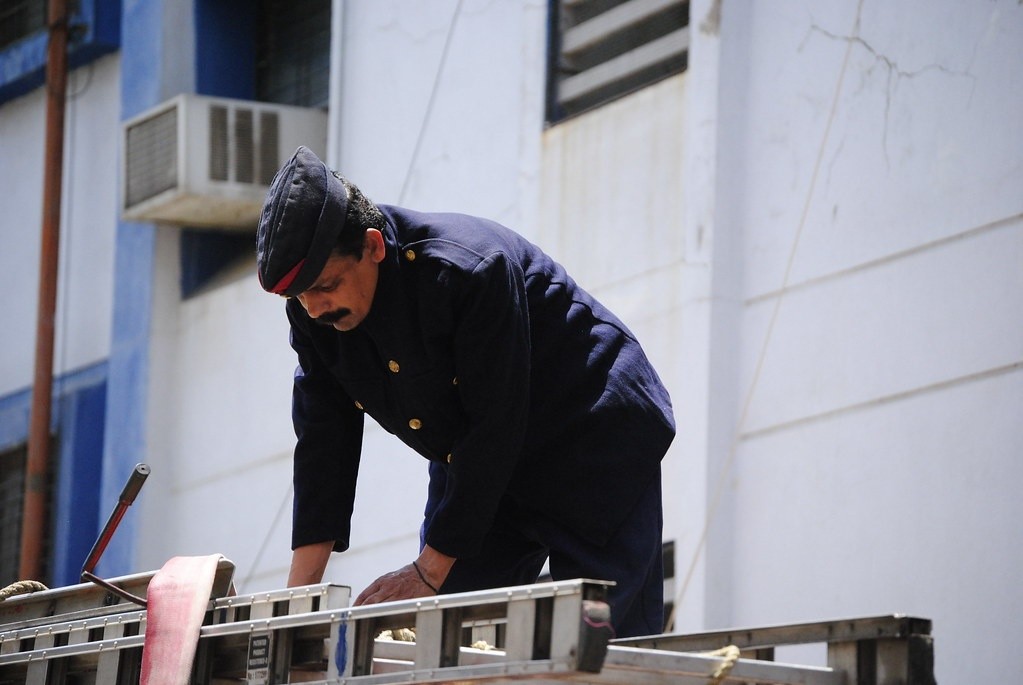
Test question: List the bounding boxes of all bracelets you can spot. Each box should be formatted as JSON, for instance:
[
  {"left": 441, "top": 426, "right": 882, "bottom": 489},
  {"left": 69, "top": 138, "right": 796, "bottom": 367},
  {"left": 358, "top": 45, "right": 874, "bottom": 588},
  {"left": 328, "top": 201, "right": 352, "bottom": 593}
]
[{"left": 413, "top": 561, "right": 439, "bottom": 595}]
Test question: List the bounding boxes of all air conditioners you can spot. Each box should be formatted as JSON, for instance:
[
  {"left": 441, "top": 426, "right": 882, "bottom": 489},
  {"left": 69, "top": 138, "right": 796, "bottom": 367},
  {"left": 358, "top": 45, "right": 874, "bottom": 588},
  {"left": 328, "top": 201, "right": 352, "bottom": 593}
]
[{"left": 119, "top": 94, "right": 326, "bottom": 232}]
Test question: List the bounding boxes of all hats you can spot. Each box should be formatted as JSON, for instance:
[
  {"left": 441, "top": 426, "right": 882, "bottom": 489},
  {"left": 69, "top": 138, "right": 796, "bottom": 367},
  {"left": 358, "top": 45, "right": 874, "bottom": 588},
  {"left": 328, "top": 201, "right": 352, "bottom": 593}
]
[{"left": 255, "top": 146, "right": 347, "bottom": 298}]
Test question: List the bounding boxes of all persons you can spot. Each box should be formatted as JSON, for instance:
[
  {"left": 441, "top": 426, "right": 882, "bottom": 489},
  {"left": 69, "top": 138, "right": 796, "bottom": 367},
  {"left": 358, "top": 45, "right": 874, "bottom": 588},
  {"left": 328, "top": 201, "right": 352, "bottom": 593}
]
[{"left": 258, "top": 146, "right": 675, "bottom": 639}]
